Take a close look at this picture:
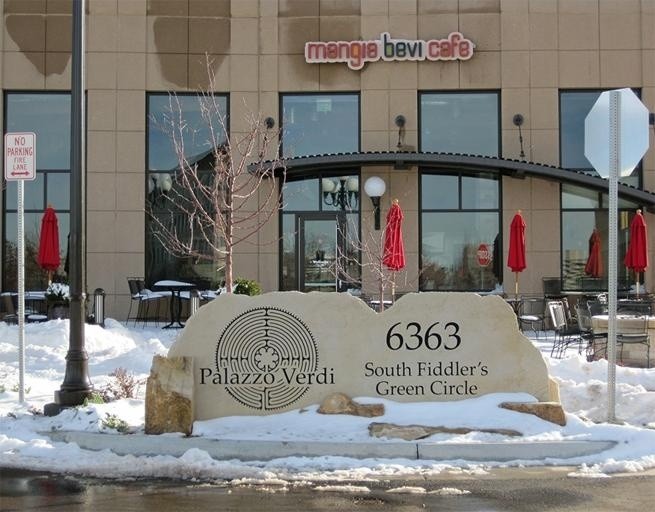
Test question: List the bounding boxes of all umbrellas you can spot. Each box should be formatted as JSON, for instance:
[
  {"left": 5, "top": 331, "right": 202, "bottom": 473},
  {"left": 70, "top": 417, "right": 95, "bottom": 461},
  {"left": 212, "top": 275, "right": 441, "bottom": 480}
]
[
  {"left": 38, "top": 203, "right": 63, "bottom": 292},
  {"left": 382, "top": 197, "right": 408, "bottom": 309},
  {"left": 583, "top": 227, "right": 604, "bottom": 280},
  {"left": 624, "top": 207, "right": 650, "bottom": 301},
  {"left": 508, "top": 208, "right": 527, "bottom": 304}
]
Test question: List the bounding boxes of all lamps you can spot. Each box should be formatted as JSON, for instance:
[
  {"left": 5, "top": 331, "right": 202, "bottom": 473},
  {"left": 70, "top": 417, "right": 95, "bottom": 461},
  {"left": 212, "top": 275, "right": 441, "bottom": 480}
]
[{"left": 363, "top": 176, "right": 387, "bottom": 230}]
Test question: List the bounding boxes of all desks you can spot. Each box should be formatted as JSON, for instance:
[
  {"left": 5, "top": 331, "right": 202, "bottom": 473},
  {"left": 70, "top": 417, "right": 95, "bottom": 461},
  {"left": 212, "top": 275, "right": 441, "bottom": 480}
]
[{"left": 370, "top": 300, "right": 392, "bottom": 309}]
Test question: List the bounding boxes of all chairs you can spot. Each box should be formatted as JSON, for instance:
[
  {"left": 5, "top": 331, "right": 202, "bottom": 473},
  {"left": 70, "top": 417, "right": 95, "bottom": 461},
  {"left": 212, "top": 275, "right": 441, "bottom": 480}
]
[
  {"left": 9, "top": 276, "right": 225, "bottom": 330},
  {"left": 504, "top": 276, "right": 655, "bottom": 368}
]
